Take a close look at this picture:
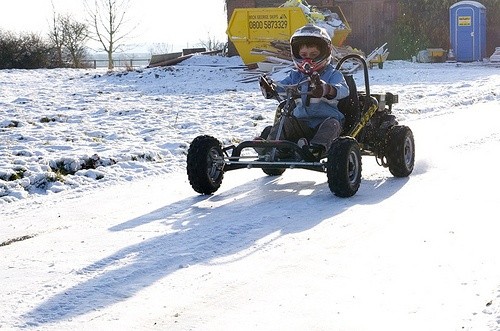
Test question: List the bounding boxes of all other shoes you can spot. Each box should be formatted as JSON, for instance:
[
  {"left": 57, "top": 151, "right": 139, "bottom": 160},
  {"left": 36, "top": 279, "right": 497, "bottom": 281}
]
[
  {"left": 252, "top": 137, "right": 268, "bottom": 155},
  {"left": 298, "top": 138, "right": 317, "bottom": 160}
]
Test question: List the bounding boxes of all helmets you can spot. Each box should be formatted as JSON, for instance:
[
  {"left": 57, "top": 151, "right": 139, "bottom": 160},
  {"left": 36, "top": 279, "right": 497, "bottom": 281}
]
[{"left": 290, "top": 24, "right": 332, "bottom": 74}]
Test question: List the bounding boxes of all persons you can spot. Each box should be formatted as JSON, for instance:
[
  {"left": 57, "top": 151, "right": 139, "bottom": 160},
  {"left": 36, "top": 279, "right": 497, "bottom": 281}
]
[{"left": 252, "top": 23, "right": 350, "bottom": 163}]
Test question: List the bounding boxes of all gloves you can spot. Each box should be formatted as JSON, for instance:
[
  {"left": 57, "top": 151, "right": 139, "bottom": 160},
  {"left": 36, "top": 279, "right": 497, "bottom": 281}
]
[
  {"left": 306, "top": 73, "right": 330, "bottom": 99},
  {"left": 258, "top": 73, "right": 277, "bottom": 99}
]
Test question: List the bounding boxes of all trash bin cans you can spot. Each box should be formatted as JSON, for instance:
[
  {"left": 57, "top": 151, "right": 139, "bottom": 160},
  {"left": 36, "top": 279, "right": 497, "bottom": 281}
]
[{"left": 226, "top": 0, "right": 352, "bottom": 68}]
